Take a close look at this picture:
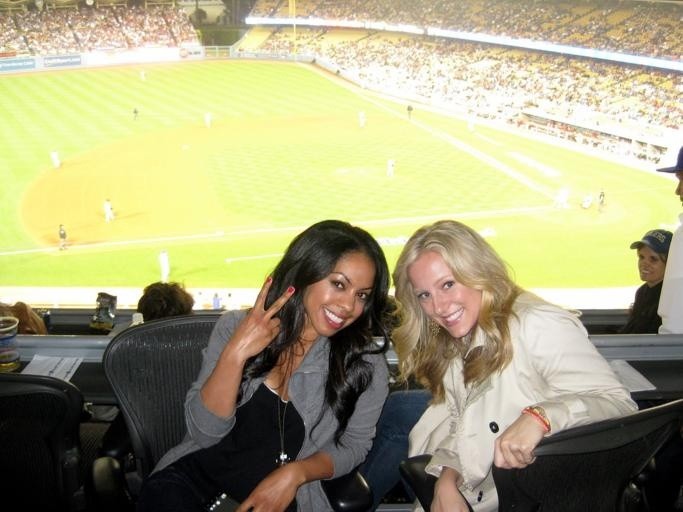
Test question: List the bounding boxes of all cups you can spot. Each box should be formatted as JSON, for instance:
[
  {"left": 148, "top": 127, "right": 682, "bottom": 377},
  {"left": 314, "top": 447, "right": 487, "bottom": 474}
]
[{"left": 0, "top": 317, "right": 20, "bottom": 372}]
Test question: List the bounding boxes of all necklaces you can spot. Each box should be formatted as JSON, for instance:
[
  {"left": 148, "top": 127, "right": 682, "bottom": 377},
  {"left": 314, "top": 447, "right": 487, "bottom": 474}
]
[{"left": 273, "top": 351, "right": 293, "bottom": 467}]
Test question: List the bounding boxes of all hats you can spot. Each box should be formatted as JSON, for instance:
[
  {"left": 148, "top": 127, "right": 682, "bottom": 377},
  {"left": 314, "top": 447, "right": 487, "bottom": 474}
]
[
  {"left": 630, "top": 229, "right": 673, "bottom": 253},
  {"left": 656, "top": 146, "right": 683, "bottom": 172}
]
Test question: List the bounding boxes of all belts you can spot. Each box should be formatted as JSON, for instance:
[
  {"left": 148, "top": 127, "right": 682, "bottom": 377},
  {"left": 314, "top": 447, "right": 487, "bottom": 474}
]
[{"left": 178, "top": 458, "right": 241, "bottom": 511}]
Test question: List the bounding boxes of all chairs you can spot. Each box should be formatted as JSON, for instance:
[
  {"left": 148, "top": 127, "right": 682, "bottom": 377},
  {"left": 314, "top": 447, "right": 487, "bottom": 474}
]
[{"left": 0, "top": 314, "right": 683, "bottom": 512}]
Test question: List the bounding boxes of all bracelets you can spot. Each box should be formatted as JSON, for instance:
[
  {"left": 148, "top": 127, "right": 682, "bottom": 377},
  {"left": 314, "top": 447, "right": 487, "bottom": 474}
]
[{"left": 520, "top": 406, "right": 552, "bottom": 433}]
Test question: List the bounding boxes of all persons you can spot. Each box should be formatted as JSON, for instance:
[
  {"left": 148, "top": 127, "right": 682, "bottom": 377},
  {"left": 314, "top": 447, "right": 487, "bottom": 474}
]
[
  {"left": 0, "top": 0, "right": 683, "bottom": 171},
  {"left": 196, "top": 291, "right": 203, "bottom": 310},
  {"left": 211, "top": 293, "right": 220, "bottom": 308},
  {"left": 103, "top": 199, "right": 115, "bottom": 224},
  {"left": 57, "top": 224, "right": 67, "bottom": 251},
  {"left": 136, "top": 280, "right": 195, "bottom": 323},
  {"left": 654, "top": 144, "right": 683, "bottom": 335},
  {"left": 157, "top": 249, "right": 171, "bottom": 283},
  {"left": 346, "top": 217, "right": 644, "bottom": 511},
  {"left": 224, "top": 293, "right": 233, "bottom": 309},
  {"left": 385, "top": 160, "right": 397, "bottom": 179},
  {"left": 615, "top": 228, "right": 674, "bottom": 333},
  {"left": 599, "top": 192, "right": 605, "bottom": 205},
  {"left": 131, "top": 217, "right": 403, "bottom": 511}
]
